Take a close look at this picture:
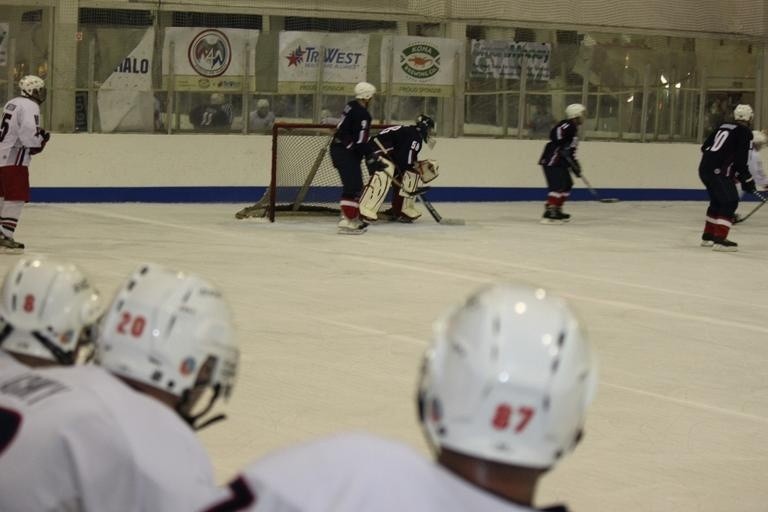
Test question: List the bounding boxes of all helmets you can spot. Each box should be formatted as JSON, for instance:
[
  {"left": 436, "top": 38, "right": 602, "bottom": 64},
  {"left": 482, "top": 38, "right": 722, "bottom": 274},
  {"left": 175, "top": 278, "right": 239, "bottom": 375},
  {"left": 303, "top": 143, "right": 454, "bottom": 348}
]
[
  {"left": 418, "top": 284, "right": 595, "bottom": 472},
  {"left": 256, "top": 99, "right": 270, "bottom": 108},
  {"left": 210, "top": 93, "right": 225, "bottom": 105},
  {"left": 92, "top": 263, "right": 240, "bottom": 430},
  {"left": 19, "top": 75, "right": 47, "bottom": 104},
  {"left": 354, "top": 82, "right": 376, "bottom": 100},
  {"left": 416, "top": 114, "right": 435, "bottom": 143},
  {"left": 734, "top": 104, "right": 754, "bottom": 122},
  {"left": 0, "top": 257, "right": 103, "bottom": 364},
  {"left": 565, "top": 104, "right": 586, "bottom": 119}
]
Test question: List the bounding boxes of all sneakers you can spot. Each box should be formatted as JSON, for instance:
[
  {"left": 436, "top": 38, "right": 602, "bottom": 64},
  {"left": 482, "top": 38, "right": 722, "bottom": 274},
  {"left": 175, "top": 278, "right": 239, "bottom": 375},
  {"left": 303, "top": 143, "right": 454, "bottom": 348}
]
[
  {"left": 338, "top": 218, "right": 370, "bottom": 230},
  {"left": 713, "top": 237, "right": 738, "bottom": 247},
  {"left": 543, "top": 207, "right": 570, "bottom": 219},
  {"left": 702, "top": 232, "right": 715, "bottom": 241},
  {"left": 0, "top": 233, "right": 24, "bottom": 249}
]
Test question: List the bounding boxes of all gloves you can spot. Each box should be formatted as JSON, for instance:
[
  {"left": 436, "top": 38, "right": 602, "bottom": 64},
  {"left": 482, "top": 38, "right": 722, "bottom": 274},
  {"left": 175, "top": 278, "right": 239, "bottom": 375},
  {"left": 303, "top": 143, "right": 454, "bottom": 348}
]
[
  {"left": 737, "top": 169, "right": 756, "bottom": 192},
  {"left": 29, "top": 129, "right": 50, "bottom": 155}
]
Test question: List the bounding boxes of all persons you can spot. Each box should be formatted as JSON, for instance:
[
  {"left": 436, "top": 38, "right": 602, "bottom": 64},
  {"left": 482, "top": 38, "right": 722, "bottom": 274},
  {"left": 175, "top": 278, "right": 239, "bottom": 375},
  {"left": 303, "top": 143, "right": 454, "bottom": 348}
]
[
  {"left": 359, "top": 114, "right": 439, "bottom": 220},
  {"left": 330, "top": 81, "right": 377, "bottom": 230},
  {"left": 0, "top": 75, "right": 50, "bottom": 248},
  {"left": 699, "top": 104, "right": 755, "bottom": 247},
  {"left": 181, "top": 284, "right": 600, "bottom": 512},
  {"left": 0, "top": 263, "right": 238, "bottom": 511},
  {"left": 154, "top": 97, "right": 161, "bottom": 130},
  {"left": 735, "top": 131, "right": 767, "bottom": 199},
  {"left": 471, "top": 42, "right": 548, "bottom": 81},
  {"left": 538, "top": 103, "right": 586, "bottom": 220},
  {"left": 0, "top": 258, "right": 103, "bottom": 387},
  {"left": 189, "top": 94, "right": 231, "bottom": 131},
  {"left": 240, "top": 100, "right": 275, "bottom": 129}
]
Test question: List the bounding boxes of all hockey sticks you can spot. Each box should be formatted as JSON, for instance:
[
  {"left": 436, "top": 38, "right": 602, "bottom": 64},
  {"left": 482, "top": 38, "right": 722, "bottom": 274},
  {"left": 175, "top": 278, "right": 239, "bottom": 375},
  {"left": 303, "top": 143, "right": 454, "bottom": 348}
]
[
  {"left": 374, "top": 139, "right": 464, "bottom": 224},
  {"left": 563, "top": 151, "right": 618, "bottom": 203}
]
